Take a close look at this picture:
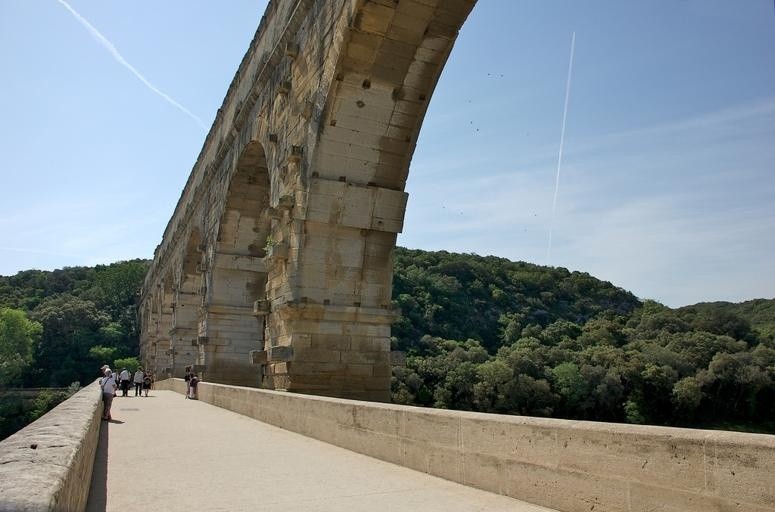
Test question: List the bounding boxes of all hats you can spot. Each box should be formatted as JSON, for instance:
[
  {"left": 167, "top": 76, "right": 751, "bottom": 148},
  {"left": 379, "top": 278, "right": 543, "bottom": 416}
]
[{"left": 100, "top": 364, "right": 109, "bottom": 370}]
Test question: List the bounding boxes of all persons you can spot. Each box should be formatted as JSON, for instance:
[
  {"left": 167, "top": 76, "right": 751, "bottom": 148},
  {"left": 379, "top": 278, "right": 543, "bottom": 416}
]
[
  {"left": 143, "top": 368, "right": 154, "bottom": 397},
  {"left": 99, "top": 364, "right": 110, "bottom": 401},
  {"left": 133, "top": 367, "right": 144, "bottom": 397},
  {"left": 185, "top": 373, "right": 194, "bottom": 399},
  {"left": 187, "top": 374, "right": 200, "bottom": 398},
  {"left": 100, "top": 367, "right": 117, "bottom": 420},
  {"left": 118, "top": 367, "right": 131, "bottom": 397},
  {"left": 110, "top": 368, "right": 119, "bottom": 397}
]
